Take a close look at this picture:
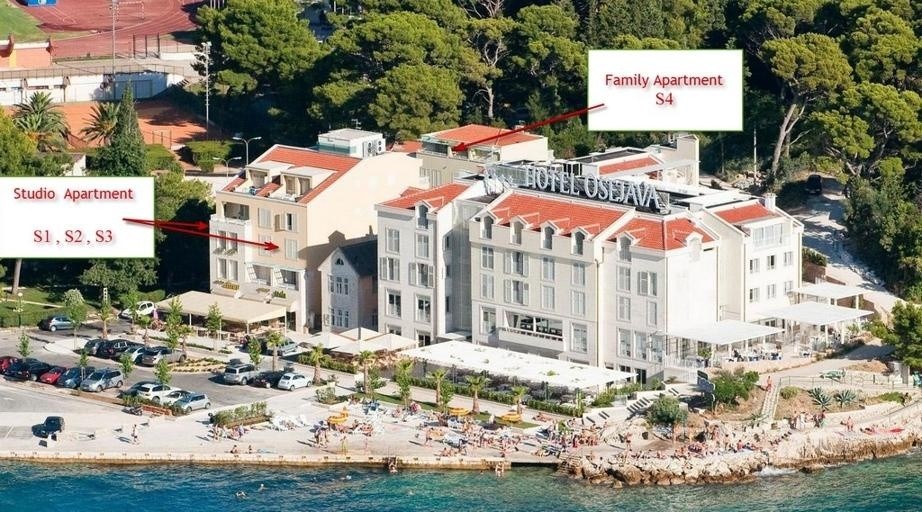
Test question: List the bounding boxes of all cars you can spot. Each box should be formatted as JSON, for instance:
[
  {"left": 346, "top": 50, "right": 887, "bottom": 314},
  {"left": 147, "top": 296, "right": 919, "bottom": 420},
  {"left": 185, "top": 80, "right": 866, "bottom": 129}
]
[
  {"left": 804, "top": 174, "right": 823, "bottom": 196},
  {"left": 121, "top": 300, "right": 154, "bottom": 319},
  {"left": 41, "top": 315, "right": 81, "bottom": 332},
  {"left": 41, "top": 416, "right": 65, "bottom": 437}
]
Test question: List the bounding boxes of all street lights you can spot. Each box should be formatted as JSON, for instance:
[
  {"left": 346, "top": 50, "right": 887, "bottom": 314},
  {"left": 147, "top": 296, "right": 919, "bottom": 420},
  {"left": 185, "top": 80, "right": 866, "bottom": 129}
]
[
  {"left": 193, "top": 41, "right": 212, "bottom": 139},
  {"left": 212, "top": 136, "right": 262, "bottom": 180}
]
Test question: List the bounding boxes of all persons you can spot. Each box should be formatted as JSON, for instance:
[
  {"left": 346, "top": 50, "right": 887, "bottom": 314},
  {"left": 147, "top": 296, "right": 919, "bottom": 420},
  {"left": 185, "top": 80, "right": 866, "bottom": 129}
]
[
  {"left": 730, "top": 348, "right": 741, "bottom": 363},
  {"left": 766, "top": 375, "right": 772, "bottom": 392},
  {"left": 212, "top": 423, "right": 220, "bottom": 440},
  {"left": 221, "top": 421, "right": 229, "bottom": 441},
  {"left": 237, "top": 423, "right": 244, "bottom": 440},
  {"left": 313, "top": 401, "right": 855, "bottom": 461},
  {"left": 131, "top": 423, "right": 140, "bottom": 444}
]
